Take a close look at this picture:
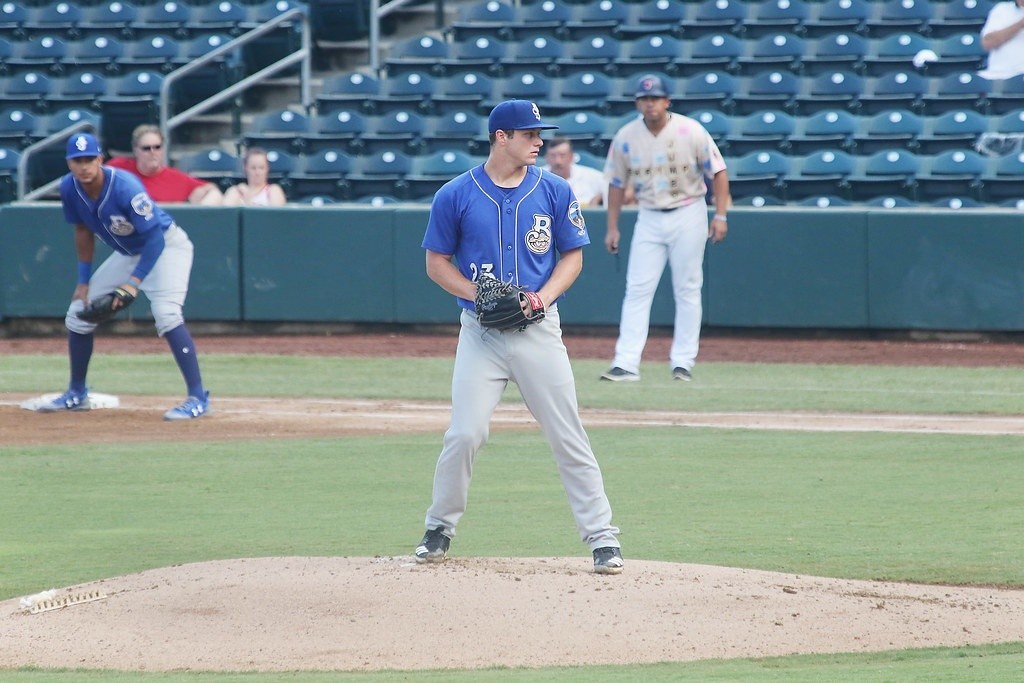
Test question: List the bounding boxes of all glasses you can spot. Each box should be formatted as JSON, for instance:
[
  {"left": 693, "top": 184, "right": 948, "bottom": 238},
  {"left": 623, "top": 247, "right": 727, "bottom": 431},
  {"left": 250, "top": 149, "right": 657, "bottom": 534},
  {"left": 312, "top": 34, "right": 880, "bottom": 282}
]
[{"left": 138, "top": 143, "right": 161, "bottom": 152}]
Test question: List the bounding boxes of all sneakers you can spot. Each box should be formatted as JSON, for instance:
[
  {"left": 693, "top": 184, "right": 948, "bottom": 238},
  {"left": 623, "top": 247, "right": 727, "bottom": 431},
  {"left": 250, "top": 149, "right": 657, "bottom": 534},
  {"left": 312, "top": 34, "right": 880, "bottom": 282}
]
[
  {"left": 672, "top": 367, "right": 690, "bottom": 380},
  {"left": 593, "top": 547, "right": 624, "bottom": 572},
  {"left": 415, "top": 527, "right": 451, "bottom": 564},
  {"left": 40, "top": 389, "right": 93, "bottom": 412},
  {"left": 601, "top": 367, "right": 639, "bottom": 381},
  {"left": 165, "top": 390, "right": 208, "bottom": 420}
]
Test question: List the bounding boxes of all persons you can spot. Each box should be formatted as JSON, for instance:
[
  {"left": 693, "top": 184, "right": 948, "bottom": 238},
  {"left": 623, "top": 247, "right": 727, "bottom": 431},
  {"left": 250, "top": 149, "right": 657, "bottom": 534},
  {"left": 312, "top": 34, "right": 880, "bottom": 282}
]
[
  {"left": 978, "top": 0, "right": 1024, "bottom": 80},
  {"left": 413, "top": 99, "right": 626, "bottom": 573},
  {"left": 38, "top": 135, "right": 209, "bottom": 419},
  {"left": 107, "top": 123, "right": 225, "bottom": 203},
  {"left": 703, "top": 174, "right": 733, "bottom": 207},
  {"left": 224, "top": 148, "right": 285, "bottom": 205},
  {"left": 542, "top": 139, "right": 603, "bottom": 206},
  {"left": 600, "top": 75, "right": 729, "bottom": 381}
]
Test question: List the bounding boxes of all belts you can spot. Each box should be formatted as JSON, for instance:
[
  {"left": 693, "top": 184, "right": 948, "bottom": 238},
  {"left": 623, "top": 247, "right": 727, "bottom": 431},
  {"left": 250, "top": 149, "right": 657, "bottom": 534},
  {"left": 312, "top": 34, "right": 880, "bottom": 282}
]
[{"left": 651, "top": 206, "right": 676, "bottom": 212}]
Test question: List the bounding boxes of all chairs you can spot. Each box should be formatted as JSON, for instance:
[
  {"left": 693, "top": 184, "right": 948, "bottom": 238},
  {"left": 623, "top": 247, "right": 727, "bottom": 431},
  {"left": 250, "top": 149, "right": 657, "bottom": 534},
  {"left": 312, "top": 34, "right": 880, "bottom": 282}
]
[{"left": 0, "top": 0, "right": 1024, "bottom": 209}]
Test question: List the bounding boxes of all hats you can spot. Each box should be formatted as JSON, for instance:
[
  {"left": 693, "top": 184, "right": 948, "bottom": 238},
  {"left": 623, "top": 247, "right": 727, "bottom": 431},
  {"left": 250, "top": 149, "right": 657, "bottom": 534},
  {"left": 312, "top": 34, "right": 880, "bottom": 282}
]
[
  {"left": 489, "top": 98, "right": 559, "bottom": 134},
  {"left": 635, "top": 75, "right": 666, "bottom": 101},
  {"left": 65, "top": 133, "right": 101, "bottom": 160}
]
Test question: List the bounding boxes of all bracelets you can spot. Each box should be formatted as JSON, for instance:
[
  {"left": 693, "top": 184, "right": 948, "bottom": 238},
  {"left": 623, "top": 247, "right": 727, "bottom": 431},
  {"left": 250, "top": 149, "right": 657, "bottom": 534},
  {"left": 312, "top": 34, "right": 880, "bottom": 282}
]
[
  {"left": 126, "top": 280, "right": 140, "bottom": 287},
  {"left": 714, "top": 214, "right": 726, "bottom": 221}
]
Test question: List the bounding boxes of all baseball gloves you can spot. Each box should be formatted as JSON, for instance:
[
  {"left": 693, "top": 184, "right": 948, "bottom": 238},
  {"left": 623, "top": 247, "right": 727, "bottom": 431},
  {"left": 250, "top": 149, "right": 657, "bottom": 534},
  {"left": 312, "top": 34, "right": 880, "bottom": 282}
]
[
  {"left": 475, "top": 273, "right": 547, "bottom": 332},
  {"left": 74, "top": 287, "right": 134, "bottom": 323}
]
